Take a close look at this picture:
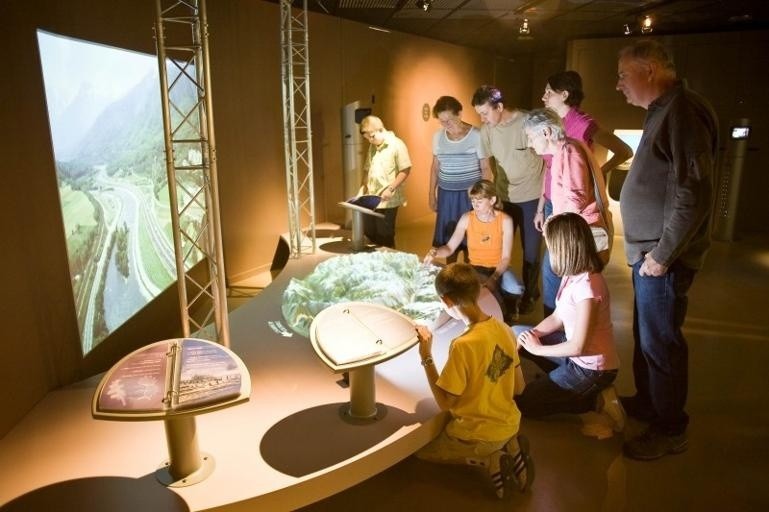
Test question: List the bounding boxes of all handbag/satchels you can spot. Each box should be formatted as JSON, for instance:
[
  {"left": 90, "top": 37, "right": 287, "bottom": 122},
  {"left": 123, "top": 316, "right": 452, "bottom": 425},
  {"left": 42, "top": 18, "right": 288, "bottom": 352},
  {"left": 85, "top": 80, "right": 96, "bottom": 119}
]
[{"left": 589, "top": 226, "right": 610, "bottom": 266}]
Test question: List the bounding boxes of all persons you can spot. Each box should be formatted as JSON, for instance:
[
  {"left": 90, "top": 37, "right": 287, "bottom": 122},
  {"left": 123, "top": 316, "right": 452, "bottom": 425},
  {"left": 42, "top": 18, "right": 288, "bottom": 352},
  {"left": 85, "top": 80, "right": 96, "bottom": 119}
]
[
  {"left": 357, "top": 115, "right": 412, "bottom": 249},
  {"left": 615, "top": 37, "right": 721, "bottom": 459},
  {"left": 510, "top": 212, "right": 628, "bottom": 435},
  {"left": 521, "top": 105, "right": 614, "bottom": 272},
  {"left": 427, "top": 96, "right": 494, "bottom": 264},
  {"left": 424, "top": 179, "right": 526, "bottom": 326},
  {"left": 413, "top": 261, "right": 536, "bottom": 501},
  {"left": 532, "top": 70, "right": 634, "bottom": 319},
  {"left": 472, "top": 84, "right": 548, "bottom": 314}
]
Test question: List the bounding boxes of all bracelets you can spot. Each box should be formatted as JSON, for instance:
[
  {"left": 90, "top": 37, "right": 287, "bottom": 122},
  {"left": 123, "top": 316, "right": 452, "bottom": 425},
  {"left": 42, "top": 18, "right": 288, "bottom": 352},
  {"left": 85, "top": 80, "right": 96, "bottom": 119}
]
[{"left": 492, "top": 274, "right": 499, "bottom": 283}]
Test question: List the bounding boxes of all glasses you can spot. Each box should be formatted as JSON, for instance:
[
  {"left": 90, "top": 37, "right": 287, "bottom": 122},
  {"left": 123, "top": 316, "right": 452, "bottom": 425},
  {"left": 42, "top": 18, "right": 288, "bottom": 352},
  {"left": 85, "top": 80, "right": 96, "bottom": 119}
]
[{"left": 441, "top": 120, "right": 455, "bottom": 127}]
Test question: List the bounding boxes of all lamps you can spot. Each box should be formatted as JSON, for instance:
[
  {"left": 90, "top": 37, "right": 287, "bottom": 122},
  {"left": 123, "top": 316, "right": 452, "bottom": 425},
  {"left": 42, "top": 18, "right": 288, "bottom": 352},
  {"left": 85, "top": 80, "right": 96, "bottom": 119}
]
[{"left": 606, "top": 129, "right": 644, "bottom": 202}]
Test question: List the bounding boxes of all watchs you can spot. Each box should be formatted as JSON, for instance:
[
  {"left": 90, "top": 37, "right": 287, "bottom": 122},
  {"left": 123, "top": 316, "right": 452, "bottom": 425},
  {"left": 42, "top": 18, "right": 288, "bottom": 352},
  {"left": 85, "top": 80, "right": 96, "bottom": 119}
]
[
  {"left": 388, "top": 186, "right": 394, "bottom": 192},
  {"left": 422, "top": 356, "right": 435, "bottom": 367}
]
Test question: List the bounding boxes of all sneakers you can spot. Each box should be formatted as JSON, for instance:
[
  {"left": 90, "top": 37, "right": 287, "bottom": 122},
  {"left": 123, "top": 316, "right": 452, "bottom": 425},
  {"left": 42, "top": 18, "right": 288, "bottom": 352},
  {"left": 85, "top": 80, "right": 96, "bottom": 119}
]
[
  {"left": 487, "top": 451, "right": 514, "bottom": 500},
  {"left": 580, "top": 410, "right": 613, "bottom": 439},
  {"left": 600, "top": 384, "right": 627, "bottom": 433},
  {"left": 505, "top": 434, "right": 535, "bottom": 493},
  {"left": 505, "top": 314, "right": 520, "bottom": 326},
  {"left": 520, "top": 289, "right": 540, "bottom": 314},
  {"left": 623, "top": 428, "right": 690, "bottom": 461},
  {"left": 618, "top": 394, "right": 649, "bottom": 421}
]
